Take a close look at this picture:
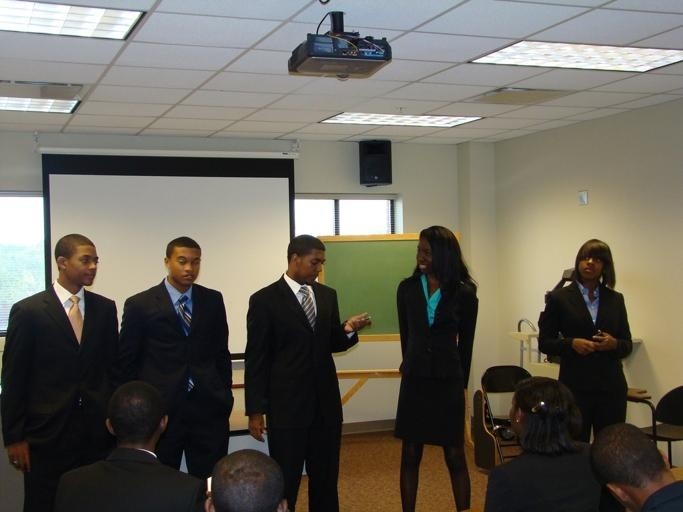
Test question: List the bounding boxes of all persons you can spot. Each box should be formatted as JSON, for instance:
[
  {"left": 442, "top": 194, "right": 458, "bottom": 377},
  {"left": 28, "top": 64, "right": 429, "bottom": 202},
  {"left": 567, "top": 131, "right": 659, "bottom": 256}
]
[
  {"left": 118, "top": 236, "right": 234, "bottom": 478},
  {"left": 484, "top": 377, "right": 625, "bottom": 512},
  {"left": 591, "top": 423, "right": 683, "bottom": 512},
  {"left": 392, "top": 225, "right": 479, "bottom": 511},
  {"left": 204, "top": 448, "right": 291, "bottom": 512},
  {"left": 54, "top": 381, "right": 207, "bottom": 512},
  {"left": 243, "top": 235, "right": 374, "bottom": 511},
  {"left": 0, "top": 233, "right": 119, "bottom": 512},
  {"left": 539, "top": 238, "right": 632, "bottom": 445}
]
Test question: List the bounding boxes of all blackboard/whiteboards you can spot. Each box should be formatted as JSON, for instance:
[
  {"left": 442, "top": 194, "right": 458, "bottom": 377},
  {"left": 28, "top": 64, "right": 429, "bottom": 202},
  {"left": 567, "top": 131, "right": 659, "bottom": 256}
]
[{"left": 318, "top": 232, "right": 460, "bottom": 342}]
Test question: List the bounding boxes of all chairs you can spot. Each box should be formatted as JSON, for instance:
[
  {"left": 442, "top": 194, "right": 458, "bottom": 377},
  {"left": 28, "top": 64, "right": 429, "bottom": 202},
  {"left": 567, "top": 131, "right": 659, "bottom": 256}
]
[
  {"left": 625, "top": 384, "right": 682, "bottom": 469},
  {"left": 481, "top": 365, "right": 531, "bottom": 466}
]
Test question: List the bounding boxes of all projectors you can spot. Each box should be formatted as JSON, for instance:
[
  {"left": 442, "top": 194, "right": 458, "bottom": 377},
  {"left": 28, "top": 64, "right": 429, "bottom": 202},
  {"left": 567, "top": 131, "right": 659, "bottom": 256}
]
[{"left": 286, "top": 31, "right": 393, "bottom": 81}]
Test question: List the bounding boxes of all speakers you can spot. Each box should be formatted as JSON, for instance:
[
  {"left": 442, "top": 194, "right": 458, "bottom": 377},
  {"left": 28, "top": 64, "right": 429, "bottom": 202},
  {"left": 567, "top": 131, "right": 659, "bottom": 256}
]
[{"left": 358, "top": 140, "right": 394, "bottom": 187}]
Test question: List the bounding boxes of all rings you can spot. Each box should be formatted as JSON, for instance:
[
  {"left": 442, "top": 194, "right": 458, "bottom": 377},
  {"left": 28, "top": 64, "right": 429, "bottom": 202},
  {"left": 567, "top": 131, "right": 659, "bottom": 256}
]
[{"left": 12, "top": 459, "right": 19, "bottom": 466}]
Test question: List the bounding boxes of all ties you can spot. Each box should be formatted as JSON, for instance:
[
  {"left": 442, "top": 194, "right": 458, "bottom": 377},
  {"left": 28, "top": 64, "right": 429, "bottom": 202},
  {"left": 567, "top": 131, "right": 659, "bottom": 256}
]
[
  {"left": 299, "top": 287, "right": 315, "bottom": 333},
  {"left": 69, "top": 296, "right": 83, "bottom": 343},
  {"left": 178, "top": 295, "right": 193, "bottom": 393}
]
